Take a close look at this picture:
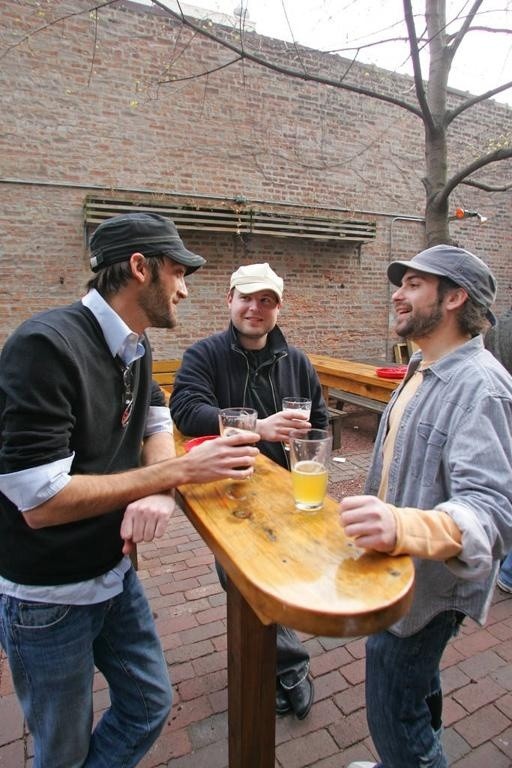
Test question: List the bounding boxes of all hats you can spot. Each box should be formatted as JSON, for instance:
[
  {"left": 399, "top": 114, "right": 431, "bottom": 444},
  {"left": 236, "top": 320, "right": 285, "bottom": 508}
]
[
  {"left": 230, "top": 262, "right": 285, "bottom": 304},
  {"left": 388, "top": 243, "right": 498, "bottom": 327},
  {"left": 88, "top": 213, "right": 207, "bottom": 277}
]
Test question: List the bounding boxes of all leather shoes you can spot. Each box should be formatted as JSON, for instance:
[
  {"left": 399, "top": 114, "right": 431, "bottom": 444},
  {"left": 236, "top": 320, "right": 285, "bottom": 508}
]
[{"left": 274, "top": 667, "right": 314, "bottom": 719}]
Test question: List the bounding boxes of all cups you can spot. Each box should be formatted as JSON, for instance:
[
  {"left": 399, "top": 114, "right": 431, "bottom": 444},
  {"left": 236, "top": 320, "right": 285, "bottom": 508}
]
[{"left": 219, "top": 398, "right": 333, "bottom": 512}]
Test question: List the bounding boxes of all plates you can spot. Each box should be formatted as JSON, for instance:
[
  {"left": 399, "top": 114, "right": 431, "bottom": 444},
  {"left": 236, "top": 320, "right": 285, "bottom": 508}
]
[
  {"left": 183, "top": 435, "right": 220, "bottom": 453},
  {"left": 377, "top": 368, "right": 408, "bottom": 378}
]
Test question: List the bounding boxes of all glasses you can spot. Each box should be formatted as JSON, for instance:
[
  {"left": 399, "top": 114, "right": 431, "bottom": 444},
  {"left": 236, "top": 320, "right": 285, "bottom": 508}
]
[{"left": 119, "top": 365, "right": 135, "bottom": 426}]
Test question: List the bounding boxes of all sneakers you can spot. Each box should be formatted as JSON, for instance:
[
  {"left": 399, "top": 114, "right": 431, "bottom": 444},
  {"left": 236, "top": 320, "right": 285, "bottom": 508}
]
[
  {"left": 496, "top": 575, "right": 512, "bottom": 593},
  {"left": 342, "top": 762, "right": 378, "bottom": 768}
]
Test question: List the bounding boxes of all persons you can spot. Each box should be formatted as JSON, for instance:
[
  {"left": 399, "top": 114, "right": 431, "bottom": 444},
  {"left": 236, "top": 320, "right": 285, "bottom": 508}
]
[
  {"left": 336, "top": 245, "right": 512, "bottom": 766},
  {"left": 482, "top": 282, "right": 512, "bottom": 597},
  {"left": 168, "top": 263, "right": 330, "bottom": 720},
  {"left": 1, "top": 212, "right": 261, "bottom": 767}
]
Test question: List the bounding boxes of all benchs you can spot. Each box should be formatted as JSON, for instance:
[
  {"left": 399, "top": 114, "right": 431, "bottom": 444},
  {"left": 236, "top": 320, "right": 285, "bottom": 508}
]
[{"left": 152, "top": 360, "right": 348, "bottom": 449}]
